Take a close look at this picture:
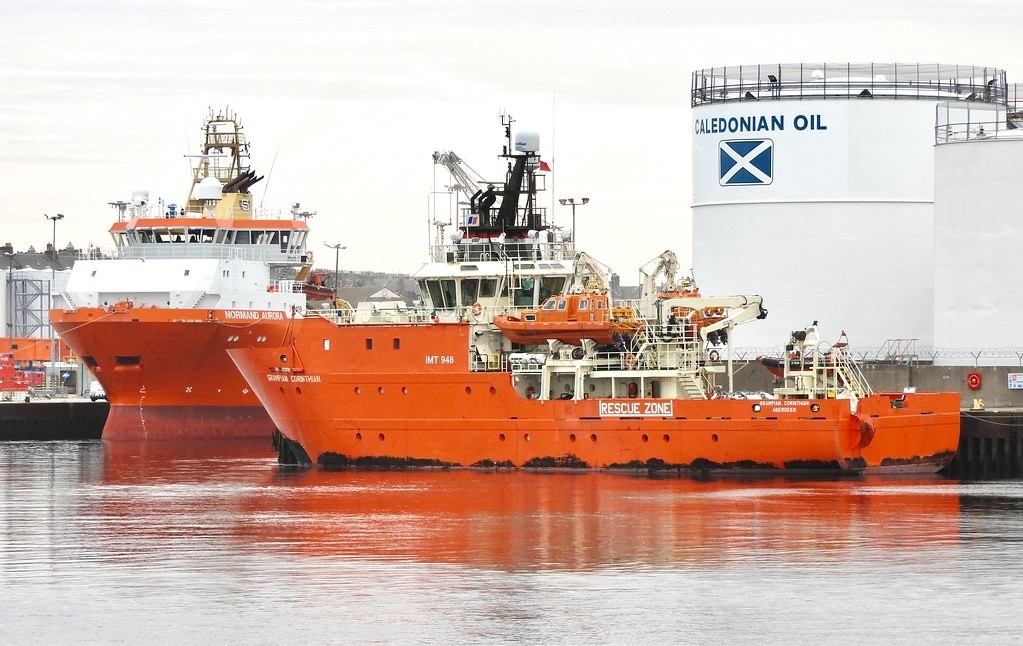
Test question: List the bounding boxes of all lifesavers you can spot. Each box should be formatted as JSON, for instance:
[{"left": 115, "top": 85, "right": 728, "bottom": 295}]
[
  {"left": 714, "top": 307, "right": 724, "bottom": 315},
  {"left": 709, "top": 349, "right": 719, "bottom": 362},
  {"left": 472, "top": 302, "right": 482, "bottom": 316},
  {"left": 968, "top": 375, "right": 980, "bottom": 387},
  {"left": 625, "top": 354, "right": 637, "bottom": 366},
  {"left": 525, "top": 386, "right": 535, "bottom": 399},
  {"left": 705, "top": 306, "right": 714, "bottom": 316}
]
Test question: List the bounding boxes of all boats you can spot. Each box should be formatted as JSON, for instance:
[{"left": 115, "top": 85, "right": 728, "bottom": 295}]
[
  {"left": 488, "top": 290, "right": 645, "bottom": 344},
  {"left": 208, "top": 107, "right": 963, "bottom": 478},
  {"left": 49, "top": 107, "right": 355, "bottom": 414},
  {"left": 366, "top": 108, "right": 619, "bottom": 382},
  {"left": 612, "top": 276, "right": 729, "bottom": 345},
  {"left": 755, "top": 328, "right": 859, "bottom": 383}
]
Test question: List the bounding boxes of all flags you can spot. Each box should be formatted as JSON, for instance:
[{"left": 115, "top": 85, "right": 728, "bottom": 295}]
[{"left": 540, "top": 160, "right": 551, "bottom": 172}]
